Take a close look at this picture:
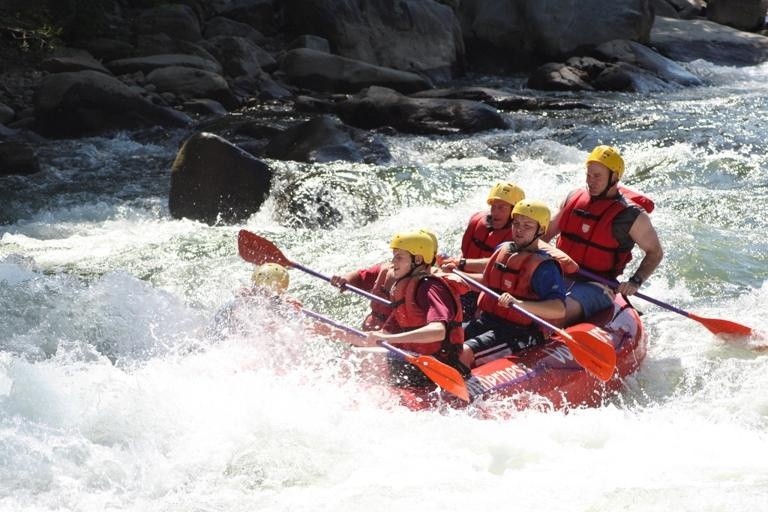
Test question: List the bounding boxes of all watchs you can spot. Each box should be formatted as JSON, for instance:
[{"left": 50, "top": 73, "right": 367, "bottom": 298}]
[{"left": 629, "top": 274, "right": 641, "bottom": 287}]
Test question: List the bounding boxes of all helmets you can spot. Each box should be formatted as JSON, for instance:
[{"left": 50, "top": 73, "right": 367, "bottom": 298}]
[
  {"left": 486, "top": 182, "right": 525, "bottom": 207},
  {"left": 249, "top": 264, "right": 291, "bottom": 296},
  {"left": 418, "top": 229, "right": 439, "bottom": 254},
  {"left": 389, "top": 232, "right": 435, "bottom": 266},
  {"left": 510, "top": 199, "right": 551, "bottom": 238},
  {"left": 584, "top": 146, "right": 626, "bottom": 181}
]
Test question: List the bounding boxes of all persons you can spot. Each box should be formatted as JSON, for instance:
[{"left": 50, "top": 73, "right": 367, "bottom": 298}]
[
  {"left": 200, "top": 263, "right": 303, "bottom": 345},
  {"left": 436, "top": 182, "right": 525, "bottom": 323},
  {"left": 314, "top": 231, "right": 474, "bottom": 386},
  {"left": 539, "top": 145, "right": 663, "bottom": 327},
  {"left": 441, "top": 199, "right": 567, "bottom": 370},
  {"left": 331, "top": 229, "right": 471, "bottom": 331}
]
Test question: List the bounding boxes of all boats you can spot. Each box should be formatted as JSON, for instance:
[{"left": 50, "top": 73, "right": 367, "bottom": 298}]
[{"left": 348, "top": 291, "right": 646, "bottom": 420}]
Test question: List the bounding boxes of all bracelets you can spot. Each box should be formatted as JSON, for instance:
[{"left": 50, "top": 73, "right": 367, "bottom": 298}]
[{"left": 458, "top": 258, "right": 466, "bottom": 270}]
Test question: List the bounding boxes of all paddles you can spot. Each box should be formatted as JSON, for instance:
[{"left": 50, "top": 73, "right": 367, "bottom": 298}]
[
  {"left": 299, "top": 308, "right": 471, "bottom": 402},
  {"left": 238, "top": 229, "right": 394, "bottom": 307},
  {"left": 451, "top": 267, "right": 618, "bottom": 383},
  {"left": 577, "top": 270, "right": 767, "bottom": 353}
]
